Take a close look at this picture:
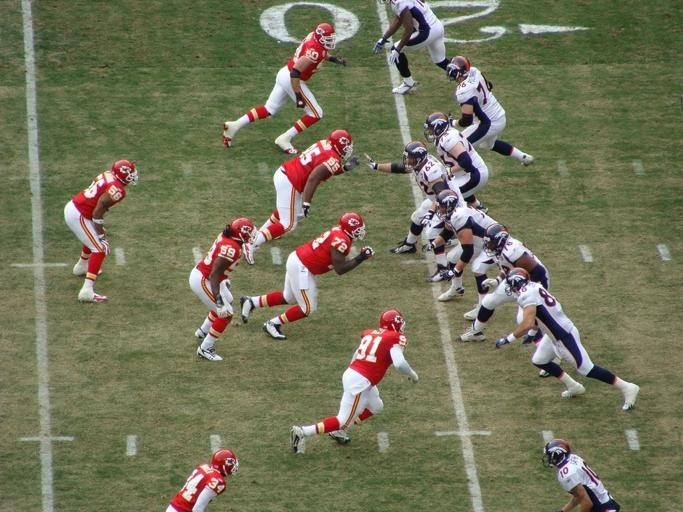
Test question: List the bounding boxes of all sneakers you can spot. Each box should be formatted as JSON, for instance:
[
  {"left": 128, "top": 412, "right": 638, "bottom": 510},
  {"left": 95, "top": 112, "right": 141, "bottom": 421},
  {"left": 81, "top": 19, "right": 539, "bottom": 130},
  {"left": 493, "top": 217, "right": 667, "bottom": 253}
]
[
  {"left": 71, "top": 264, "right": 108, "bottom": 303},
  {"left": 243, "top": 243, "right": 255, "bottom": 265},
  {"left": 622, "top": 383, "right": 639, "bottom": 410},
  {"left": 221, "top": 120, "right": 237, "bottom": 149},
  {"left": 521, "top": 154, "right": 533, "bottom": 167},
  {"left": 456, "top": 305, "right": 490, "bottom": 343},
  {"left": 392, "top": 80, "right": 418, "bottom": 95},
  {"left": 422, "top": 238, "right": 465, "bottom": 302},
  {"left": 391, "top": 240, "right": 417, "bottom": 255},
  {"left": 288, "top": 424, "right": 305, "bottom": 454},
  {"left": 239, "top": 294, "right": 255, "bottom": 324},
  {"left": 194, "top": 325, "right": 223, "bottom": 361},
  {"left": 328, "top": 428, "right": 351, "bottom": 444},
  {"left": 274, "top": 133, "right": 300, "bottom": 155},
  {"left": 561, "top": 381, "right": 585, "bottom": 399},
  {"left": 261, "top": 320, "right": 288, "bottom": 338},
  {"left": 538, "top": 357, "right": 561, "bottom": 378}
]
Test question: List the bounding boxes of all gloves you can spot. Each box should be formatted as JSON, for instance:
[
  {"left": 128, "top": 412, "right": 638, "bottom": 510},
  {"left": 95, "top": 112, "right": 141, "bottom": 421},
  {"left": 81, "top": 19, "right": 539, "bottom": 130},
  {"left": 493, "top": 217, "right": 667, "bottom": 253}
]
[
  {"left": 362, "top": 151, "right": 380, "bottom": 170},
  {"left": 481, "top": 277, "right": 501, "bottom": 288},
  {"left": 213, "top": 293, "right": 231, "bottom": 320},
  {"left": 302, "top": 201, "right": 312, "bottom": 217},
  {"left": 496, "top": 332, "right": 517, "bottom": 347},
  {"left": 328, "top": 56, "right": 348, "bottom": 66},
  {"left": 371, "top": 37, "right": 387, "bottom": 56},
  {"left": 342, "top": 155, "right": 360, "bottom": 173},
  {"left": 418, "top": 208, "right": 435, "bottom": 227},
  {"left": 98, "top": 231, "right": 112, "bottom": 258},
  {"left": 294, "top": 92, "right": 307, "bottom": 109},
  {"left": 387, "top": 46, "right": 402, "bottom": 65},
  {"left": 356, "top": 245, "right": 377, "bottom": 265}
]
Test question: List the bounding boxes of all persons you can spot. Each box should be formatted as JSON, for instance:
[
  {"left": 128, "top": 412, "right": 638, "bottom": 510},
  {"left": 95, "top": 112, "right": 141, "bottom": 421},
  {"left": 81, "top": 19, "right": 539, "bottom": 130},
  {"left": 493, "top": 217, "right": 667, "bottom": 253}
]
[
  {"left": 237, "top": 130, "right": 358, "bottom": 262},
  {"left": 447, "top": 54, "right": 533, "bottom": 167},
  {"left": 366, "top": 141, "right": 464, "bottom": 255},
  {"left": 419, "top": 162, "right": 468, "bottom": 283},
  {"left": 239, "top": 211, "right": 373, "bottom": 340},
  {"left": 421, "top": 190, "right": 499, "bottom": 319},
  {"left": 494, "top": 267, "right": 640, "bottom": 411},
  {"left": 456, "top": 223, "right": 561, "bottom": 377},
  {"left": 424, "top": 112, "right": 489, "bottom": 216},
  {"left": 543, "top": 439, "right": 621, "bottom": 512},
  {"left": 164, "top": 449, "right": 239, "bottom": 512},
  {"left": 373, "top": 0, "right": 477, "bottom": 93},
  {"left": 285, "top": 309, "right": 419, "bottom": 451},
  {"left": 220, "top": 20, "right": 348, "bottom": 153},
  {"left": 65, "top": 159, "right": 137, "bottom": 303},
  {"left": 189, "top": 218, "right": 255, "bottom": 361}
]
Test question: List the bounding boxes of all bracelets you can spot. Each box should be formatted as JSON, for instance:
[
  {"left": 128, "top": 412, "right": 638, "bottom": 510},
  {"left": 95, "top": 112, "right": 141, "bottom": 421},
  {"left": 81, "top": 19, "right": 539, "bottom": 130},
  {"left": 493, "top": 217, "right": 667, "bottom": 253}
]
[{"left": 506, "top": 334, "right": 517, "bottom": 343}]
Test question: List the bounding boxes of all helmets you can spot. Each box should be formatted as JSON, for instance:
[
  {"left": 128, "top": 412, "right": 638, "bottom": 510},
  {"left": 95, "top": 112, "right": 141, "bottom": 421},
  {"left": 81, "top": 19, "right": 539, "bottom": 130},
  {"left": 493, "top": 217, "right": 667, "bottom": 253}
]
[
  {"left": 483, "top": 222, "right": 508, "bottom": 257},
  {"left": 402, "top": 137, "right": 427, "bottom": 174},
  {"left": 112, "top": 158, "right": 140, "bottom": 186},
  {"left": 541, "top": 438, "right": 571, "bottom": 469},
  {"left": 505, "top": 267, "right": 528, "bottom": 295},
  {"left": 227, "top": 218, "right": 258, "bottom": 244},
  {"left": 435, "top": 189, "right": 459, "bottom": 220},
  {"left": 314, "top": 21, "right": 339, "bottom": 50},
  {"left": 210, "top": 448, "right": 239, "bottom": 479},
  {"left": 446, "top": 55, "right": 469, "bottom": 82},
  {"left": 423, "top": 111, "right": 449, "bottom": 143},
  {"left": 331, "top": 129, "right": 354, "bottom": 160},
  {"left": 340, "top": 212, "right": 365, "bottom": 240},
  {"left": 378, "top": 308, "right": 405, "bottom": 334}
]
